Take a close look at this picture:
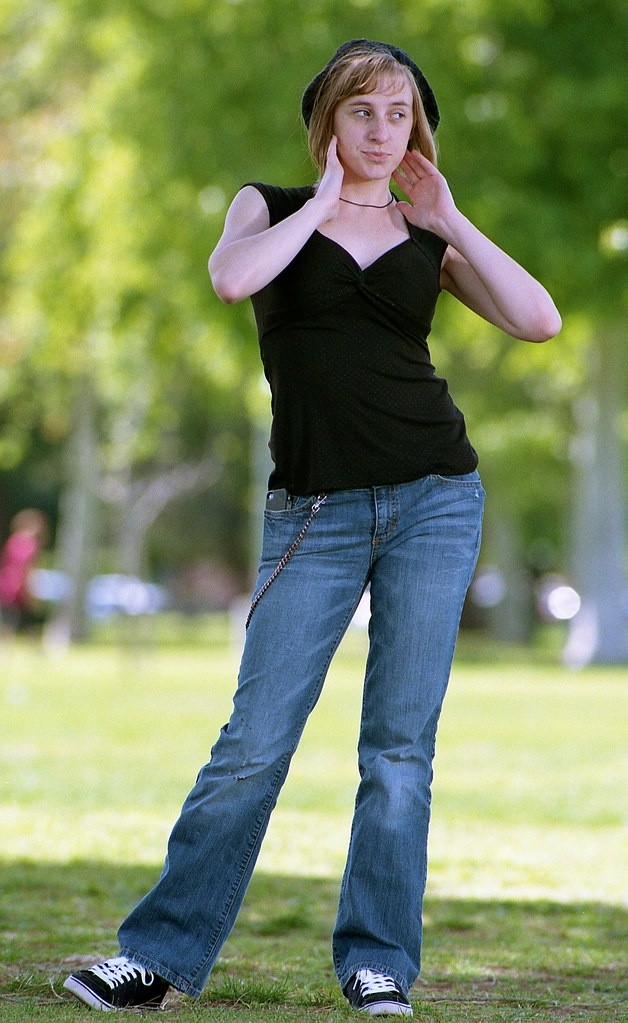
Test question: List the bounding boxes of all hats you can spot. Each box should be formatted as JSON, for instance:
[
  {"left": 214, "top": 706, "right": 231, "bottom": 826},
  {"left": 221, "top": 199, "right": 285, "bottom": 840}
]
[{"left": 301, "top": 40, "right": 440, "bottom": 152}]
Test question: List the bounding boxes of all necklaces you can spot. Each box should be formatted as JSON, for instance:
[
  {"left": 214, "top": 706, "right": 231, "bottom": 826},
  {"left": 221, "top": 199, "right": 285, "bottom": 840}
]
[{"left": 340, "top": 193, "right": 393, "bottom": 208}]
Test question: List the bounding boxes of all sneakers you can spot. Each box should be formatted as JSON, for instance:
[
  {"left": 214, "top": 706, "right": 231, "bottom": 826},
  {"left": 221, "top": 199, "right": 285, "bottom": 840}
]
[
  {"left": 346, "top": 969, "right": 414, "bottom": 1017},
  {"left": 64, "top": 956, "right": 170, "bottom": 1012}
]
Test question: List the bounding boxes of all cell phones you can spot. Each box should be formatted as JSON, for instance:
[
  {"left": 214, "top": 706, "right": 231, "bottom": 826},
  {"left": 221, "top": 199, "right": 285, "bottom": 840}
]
[{"left": 266, "top": 490, "right": 291, "bottom": 511}]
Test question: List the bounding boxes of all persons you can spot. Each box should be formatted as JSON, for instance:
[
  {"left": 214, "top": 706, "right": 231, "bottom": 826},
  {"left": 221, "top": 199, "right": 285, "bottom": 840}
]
[
  {"left": 0, "top": 508, "right": 48, "bottom": 643},
  {"left": 66, "top": 38, "right": 562, "bottom": 1013}
]
